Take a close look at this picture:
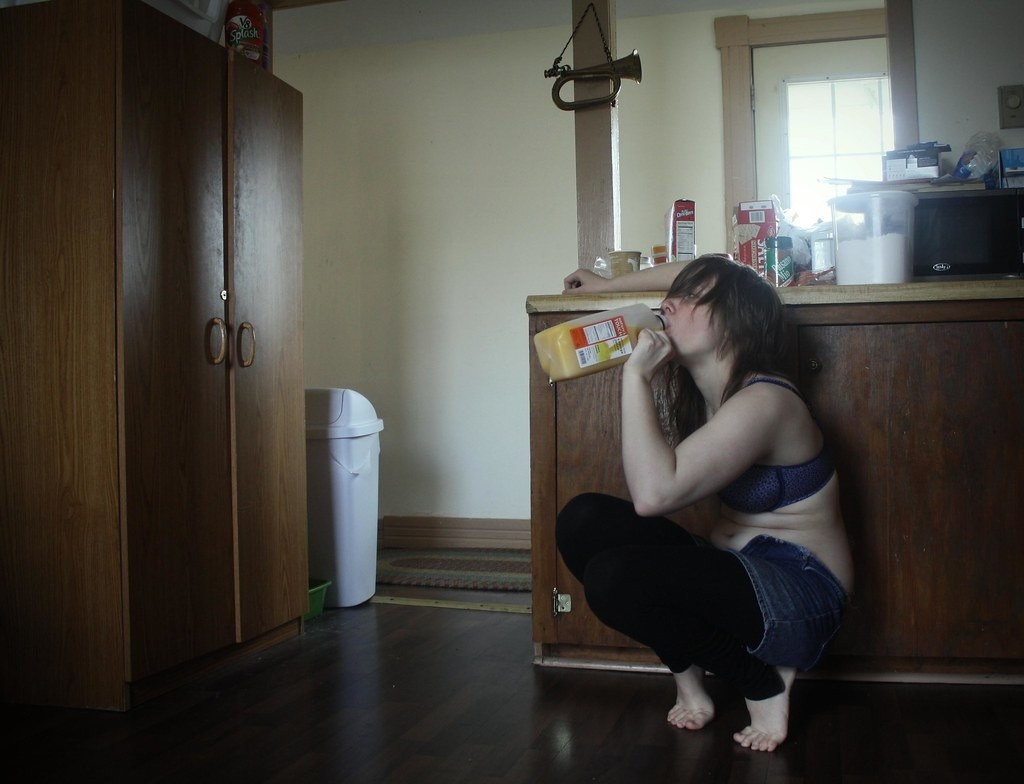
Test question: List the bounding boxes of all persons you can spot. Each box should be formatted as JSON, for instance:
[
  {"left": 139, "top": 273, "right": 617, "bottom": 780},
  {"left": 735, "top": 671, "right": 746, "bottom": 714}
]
[{"left": 553, "top": 254, "right": 857, "bottom": 753}]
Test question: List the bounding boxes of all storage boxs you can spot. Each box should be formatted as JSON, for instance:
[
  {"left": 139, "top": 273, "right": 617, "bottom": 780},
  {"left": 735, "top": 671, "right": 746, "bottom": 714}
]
[
  {"left": 665, "top": 199, "right": 696, "bottom": 262},
  {"left": 732, "top": 200, "right": 775, "bottom": 276},
  {"left": 829, "top": 191, "right": 920, "bottom": 285}
]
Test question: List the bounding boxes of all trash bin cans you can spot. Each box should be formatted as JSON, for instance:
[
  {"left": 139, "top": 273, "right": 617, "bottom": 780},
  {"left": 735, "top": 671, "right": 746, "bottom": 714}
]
[{"left": 305, "top": 387, "right": 386, "bottom": 609}]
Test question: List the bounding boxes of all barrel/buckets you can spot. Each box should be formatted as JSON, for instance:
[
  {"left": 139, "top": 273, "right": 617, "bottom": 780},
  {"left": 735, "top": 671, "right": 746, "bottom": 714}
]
[{"left": 826, "top": 191, "right": 920, "bottom": 285}]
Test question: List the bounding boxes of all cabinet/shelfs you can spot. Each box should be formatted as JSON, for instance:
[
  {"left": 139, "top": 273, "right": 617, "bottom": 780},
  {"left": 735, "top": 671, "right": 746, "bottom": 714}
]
[
  {"left": 1, "top": 0, "right": 310, "bottom": 710},
  {"left": 527, "top": 279, "right": 1024, "bottom": 685}
]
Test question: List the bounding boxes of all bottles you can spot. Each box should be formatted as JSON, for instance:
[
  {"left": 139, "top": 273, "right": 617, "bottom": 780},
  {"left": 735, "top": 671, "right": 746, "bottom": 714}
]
[
  {"left": 639, "top": 257, "right": 652, "bottom": 270},
  {"left": 764, "top": 236, "right": 795, "bottom": 288},
  {"left": 533, "top": 302, "right": 670, "bottom": 383}
]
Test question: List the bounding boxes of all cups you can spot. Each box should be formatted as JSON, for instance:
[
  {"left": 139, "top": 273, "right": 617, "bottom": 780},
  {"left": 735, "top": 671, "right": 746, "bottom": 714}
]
[{"left": 608, "top": 250, "right": 641, "bottom": 278}]
